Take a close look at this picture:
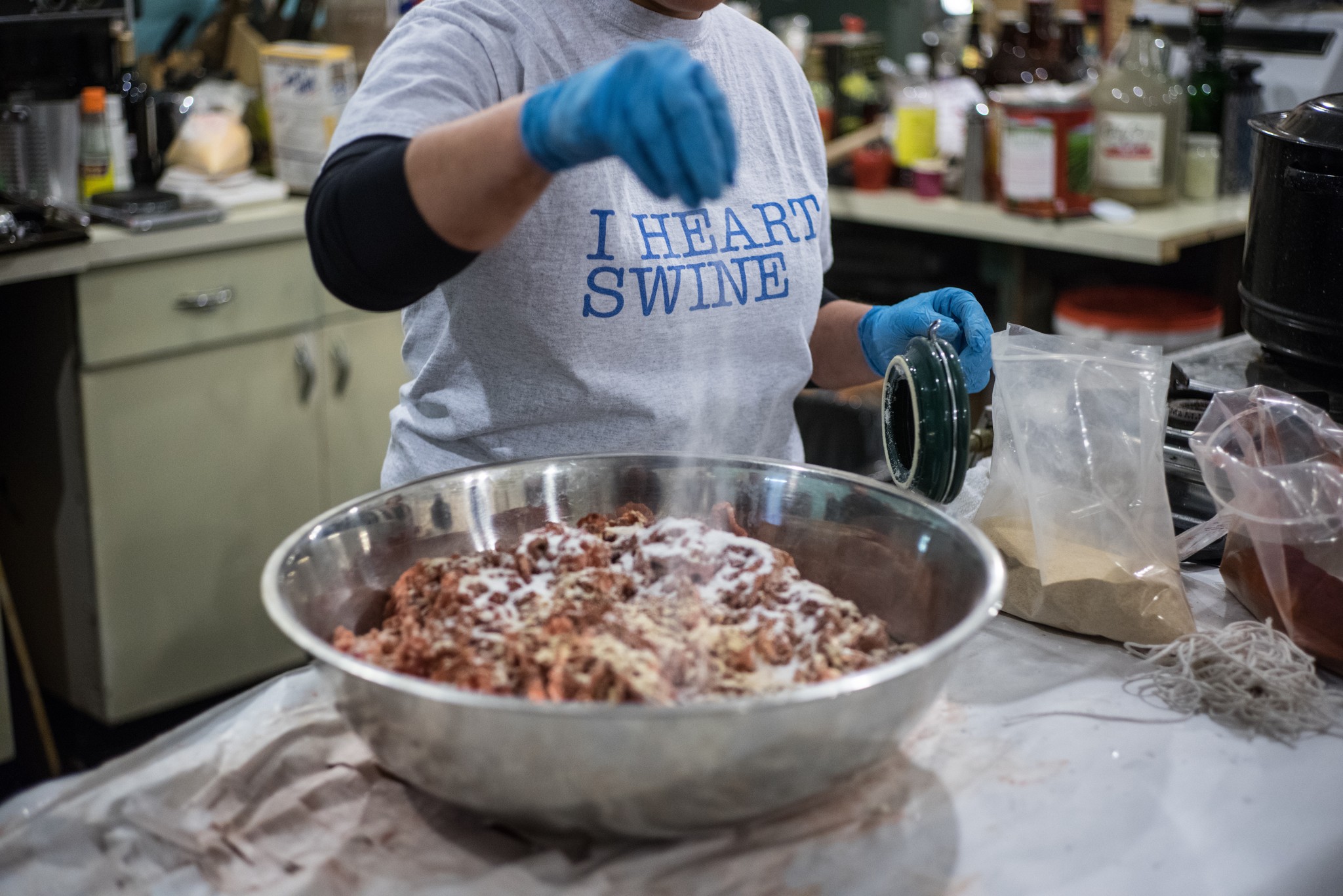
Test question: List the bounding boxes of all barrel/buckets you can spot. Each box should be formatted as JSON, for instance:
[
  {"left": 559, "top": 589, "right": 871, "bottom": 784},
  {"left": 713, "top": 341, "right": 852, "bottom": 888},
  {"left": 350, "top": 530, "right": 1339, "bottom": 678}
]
[{"left": 0, "top": 90, "right": 76, "bottom": 213}]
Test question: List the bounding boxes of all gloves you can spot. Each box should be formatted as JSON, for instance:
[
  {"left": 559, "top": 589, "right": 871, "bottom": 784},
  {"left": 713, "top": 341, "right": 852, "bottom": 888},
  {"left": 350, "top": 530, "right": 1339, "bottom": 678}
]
[
  {"left": 857, "top": 287, "right": 993, "bottom": 393},
  {"left": 520, "top": 40, "right": 737, "bottom": 207}
]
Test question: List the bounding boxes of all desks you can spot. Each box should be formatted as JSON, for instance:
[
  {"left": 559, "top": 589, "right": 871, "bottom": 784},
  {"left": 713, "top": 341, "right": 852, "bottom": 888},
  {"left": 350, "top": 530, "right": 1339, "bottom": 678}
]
[
  {"left": 831, "top": 185, "right": 1249, "bottom": 327},
  {"left": 0, "top": 464, "right": 1343, "bottom": 896}
]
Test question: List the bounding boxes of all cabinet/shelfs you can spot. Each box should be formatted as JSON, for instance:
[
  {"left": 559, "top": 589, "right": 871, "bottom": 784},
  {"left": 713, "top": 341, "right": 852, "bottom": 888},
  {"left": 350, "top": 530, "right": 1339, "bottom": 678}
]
[{"left": 0, "top": 239, "right": 413, "bottom": 723}]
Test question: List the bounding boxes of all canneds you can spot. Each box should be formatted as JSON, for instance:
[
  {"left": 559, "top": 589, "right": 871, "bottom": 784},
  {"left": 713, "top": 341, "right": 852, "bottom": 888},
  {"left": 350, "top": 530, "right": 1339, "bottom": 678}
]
[
  {"left": 817, "top": 30, "right": 888, "bottom": 139},
  {"left": 997, "top": 85, "right": 1097, "bottom": 218},
  {"left": 1183, "top": 137, "right": 1221, "bottom": 201}
]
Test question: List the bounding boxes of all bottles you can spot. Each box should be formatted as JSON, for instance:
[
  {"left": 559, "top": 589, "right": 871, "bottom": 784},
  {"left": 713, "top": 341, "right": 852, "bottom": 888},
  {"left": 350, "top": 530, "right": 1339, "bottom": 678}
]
[
  {"left": 815, "top": 1, "right": 1265, "bottom": 218},
  {"left": 80, "top": 85, "right": 113, "bottom": 207}
]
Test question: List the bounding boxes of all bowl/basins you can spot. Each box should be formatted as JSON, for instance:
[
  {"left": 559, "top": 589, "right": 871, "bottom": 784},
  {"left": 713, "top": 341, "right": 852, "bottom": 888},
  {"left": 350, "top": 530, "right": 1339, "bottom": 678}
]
[
  {"left": 1053, "top": 285, "right": 1224, "bottom": 352},
  {"left": 259, "top": 452, "right": 1009, "bottom": 839}
]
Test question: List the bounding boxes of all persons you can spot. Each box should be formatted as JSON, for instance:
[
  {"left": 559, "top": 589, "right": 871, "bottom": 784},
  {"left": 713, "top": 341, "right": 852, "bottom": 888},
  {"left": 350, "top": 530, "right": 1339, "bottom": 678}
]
[{"left": 303, "top": 0, "right": 992, "bottom": 490}]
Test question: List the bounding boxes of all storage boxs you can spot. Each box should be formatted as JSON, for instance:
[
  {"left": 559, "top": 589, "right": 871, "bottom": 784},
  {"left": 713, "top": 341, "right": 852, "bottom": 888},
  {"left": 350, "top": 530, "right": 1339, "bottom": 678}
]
[{"left": 259, "top": 42, "right": 360, "bottom": 196}]
